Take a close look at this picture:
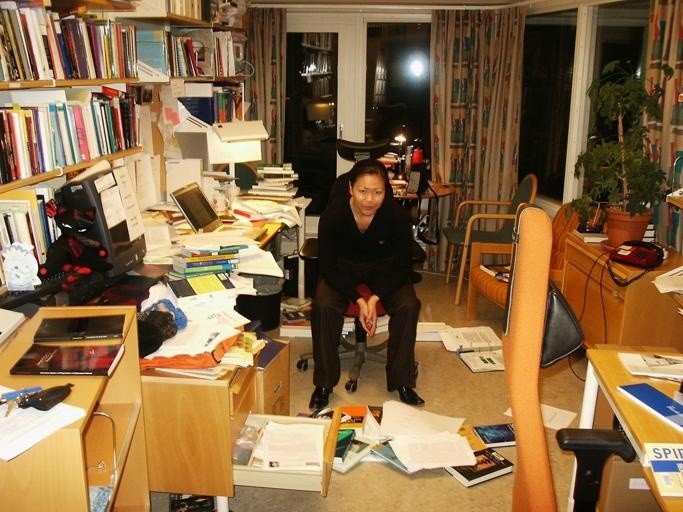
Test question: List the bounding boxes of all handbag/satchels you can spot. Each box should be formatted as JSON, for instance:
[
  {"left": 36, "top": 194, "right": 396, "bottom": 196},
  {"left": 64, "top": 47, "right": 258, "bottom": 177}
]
[
  {"left": 501, "top": 203, "right": 586, "bottom": 369},
  {"left": 405, "top": 161, "right": 441, "bottom": 247}
]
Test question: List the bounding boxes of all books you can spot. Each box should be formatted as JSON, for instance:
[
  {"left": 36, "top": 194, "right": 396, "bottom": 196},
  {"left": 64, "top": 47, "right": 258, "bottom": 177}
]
[
  {"left": 0, "top": 309, "right": 26, "bottom": 343},
  {"left": 169, "top": 0, "right": 203, "bottom": 21},
  {"left": 279, "top": 295, "right": 389, "bottom": 339},
  {"left": 140, "top": 248, "right": 267, "bottom": 379},
  {"left": 416, "top": 320, "right": 506, "bottom": 374},
  {"left": 0, "top": 85, "right": 138, "bottom": 184},
  {"left": 168, "top": 492, "right": 229, "bottom": 512},
  {"left": 232, "top": 161, "right": 300, "bottom": 224},
  {"left": 297, "top": 404, "right": 517, "bottom": 488},
  {"left": 0, "top": 181, "right": 62, "bottom": 289},
  {"left": 247, "top": 419, "right": 325, "bottom": 477},
  {"left": 169, "top": 28, "right": 237, "bottom": 77},
  {"left": 643, "top": 223, "right": 655, "bottom": 243},
  {"left": 33, "top": 313, "right": 127, "bottom": 340},
  {"left": 644, "top": 441, "right": 683, "bottom": 498},
  {"left": 9, "top": 343, "right": 126, "bottom": 376},
  {"left": 0, "top": 0, "right": 139, "bottom": 82},
  {"left": 616, "top": 382, "right": 683, "bottom": 433},
  {"left": 479, "top": 261, "right": 512, "bottom": 283},
  {"left": 618, "top": 351, "right": 683, "bottom": 379}
]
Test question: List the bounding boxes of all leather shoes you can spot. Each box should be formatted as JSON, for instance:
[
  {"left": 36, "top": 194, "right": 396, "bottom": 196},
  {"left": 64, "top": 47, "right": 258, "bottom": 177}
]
[
  {"left": 308, "top": 385, "right": 333, "bottom": 411},
  {"left": 387, "top": 381, "right": 426, "bottom": 406}
]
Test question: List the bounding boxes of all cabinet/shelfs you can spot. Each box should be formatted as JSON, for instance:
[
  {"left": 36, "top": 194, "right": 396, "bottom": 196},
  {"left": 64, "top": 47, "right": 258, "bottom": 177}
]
[
  {"left": 255, "top": 338, "right": 290, "bottom": 416},
  {"left": 299, "top": 33, "right": 338, "bottom": 101},
  {"left": 0, "top": 1, "right": 247, "bottom": 297},
  {"left": 561, "top": 231, "right": 683, "bottom": 351},
  {"left": 0, "top": 305, "right": 150, "bottom": 512},
  {"left": 141, "top": 326, "right": 342, "bottom": 512}
]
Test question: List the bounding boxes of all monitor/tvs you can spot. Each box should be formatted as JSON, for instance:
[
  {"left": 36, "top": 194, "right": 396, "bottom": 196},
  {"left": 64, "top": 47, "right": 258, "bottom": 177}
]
[{"left": 62, "top": 166, "right": 150, "bottom": 279}]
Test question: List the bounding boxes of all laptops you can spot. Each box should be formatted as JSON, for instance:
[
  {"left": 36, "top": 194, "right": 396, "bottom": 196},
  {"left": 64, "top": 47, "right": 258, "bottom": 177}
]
[{"left": 169, "top": 180, "right": 269, "bottom": 242}]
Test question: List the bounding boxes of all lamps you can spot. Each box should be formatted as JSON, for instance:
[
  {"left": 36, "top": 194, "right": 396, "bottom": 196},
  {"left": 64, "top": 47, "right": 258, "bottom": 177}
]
[
  {"left": 204, "top": 127, "right": 262, "bottom": 185},
  {"left": 307, "top": 103, "right": 332, "bottom": 120}
]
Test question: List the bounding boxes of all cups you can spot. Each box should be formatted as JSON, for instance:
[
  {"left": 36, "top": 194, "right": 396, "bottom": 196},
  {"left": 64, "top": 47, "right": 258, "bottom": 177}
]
[{"left": 213, "top": 194, "right": 231, "bottom": 212}]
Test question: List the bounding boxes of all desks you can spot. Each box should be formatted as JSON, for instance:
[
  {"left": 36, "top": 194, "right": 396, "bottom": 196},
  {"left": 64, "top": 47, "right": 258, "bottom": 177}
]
[
  {"left": 388, "top": 181, "right": 455, "bottom": 272},
  {"left": 255, "top": 196, "right": 313, "bottom": 302},
  {"left": 567, "top": 344, "right": 683, "bottom": 512}
]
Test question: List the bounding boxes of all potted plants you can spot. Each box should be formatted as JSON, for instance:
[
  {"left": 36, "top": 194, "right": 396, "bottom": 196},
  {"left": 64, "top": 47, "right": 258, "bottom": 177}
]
[{"left": 567, "top": 59, "right": 666, "bottom": 253}]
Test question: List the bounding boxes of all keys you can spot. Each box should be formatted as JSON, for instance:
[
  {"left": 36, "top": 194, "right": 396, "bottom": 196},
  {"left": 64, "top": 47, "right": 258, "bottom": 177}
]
[{"left": 4, "top": 383, "right": 72, "bottom": 416}]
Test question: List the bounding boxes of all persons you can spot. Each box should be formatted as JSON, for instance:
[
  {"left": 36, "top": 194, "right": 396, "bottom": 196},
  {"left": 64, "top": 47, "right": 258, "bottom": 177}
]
[{"left": 307, "top": 158, "right": 427, "bottom": 409}]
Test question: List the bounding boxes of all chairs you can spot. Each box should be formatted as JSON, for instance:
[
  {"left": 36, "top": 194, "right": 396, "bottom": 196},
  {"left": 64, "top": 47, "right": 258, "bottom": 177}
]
[
  {"left": 444, "top": 175, "right": 538, "bottom": 306},
  {"left": 296, "top": 138, "right": 427, "bottom": 392},
  {"left": 466, "top": 202, "right": 606, "bottom": 322}
]
